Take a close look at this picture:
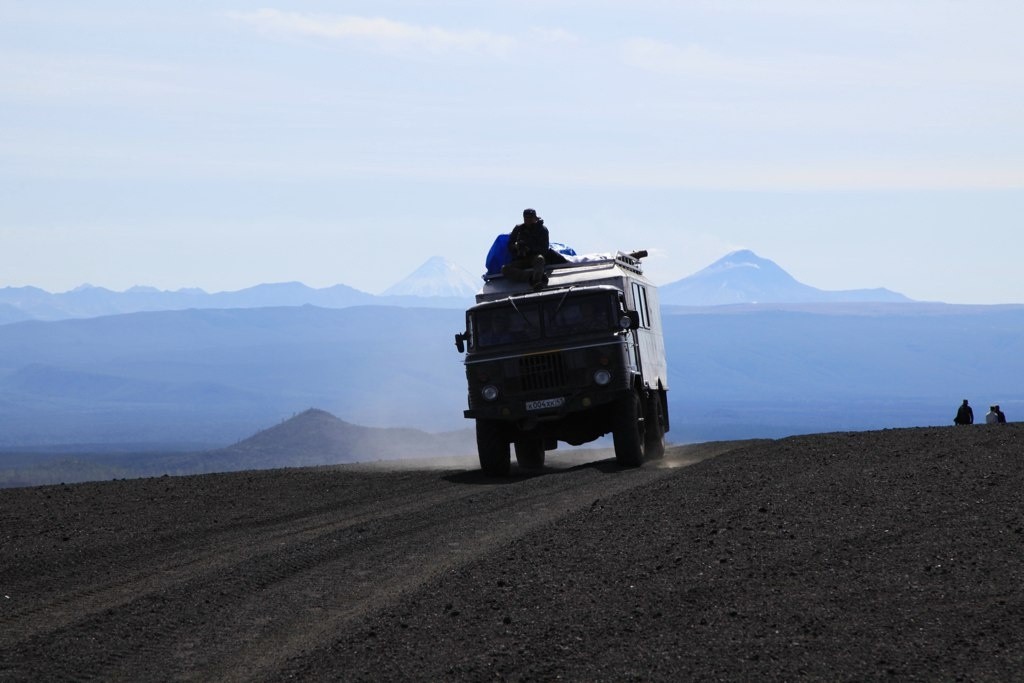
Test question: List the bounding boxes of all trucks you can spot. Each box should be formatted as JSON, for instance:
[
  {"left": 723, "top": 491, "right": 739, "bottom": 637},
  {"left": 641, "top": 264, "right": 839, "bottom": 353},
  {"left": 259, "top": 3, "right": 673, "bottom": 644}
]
[{"left": 455, "top": 250, "right": 669, "bottom": 475}]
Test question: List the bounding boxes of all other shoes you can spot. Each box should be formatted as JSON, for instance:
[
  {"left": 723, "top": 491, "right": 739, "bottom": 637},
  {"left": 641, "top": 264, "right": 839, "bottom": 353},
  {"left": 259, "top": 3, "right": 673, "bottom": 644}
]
[
  {"left": 533, "top": 273, "right": 548, "bottom": 292},
  {"left": 534, "top": 255, "right": 545, "bottom": 282}
]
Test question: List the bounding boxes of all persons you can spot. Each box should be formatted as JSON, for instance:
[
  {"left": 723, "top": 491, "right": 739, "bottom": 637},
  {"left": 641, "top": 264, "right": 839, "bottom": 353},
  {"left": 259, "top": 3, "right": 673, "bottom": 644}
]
[
  {"left": 502, "top": 209, "right": 549, "bottom": 290},
  {"left": 953, "top": 400, "right": 1006, "bottom": 427}
]
